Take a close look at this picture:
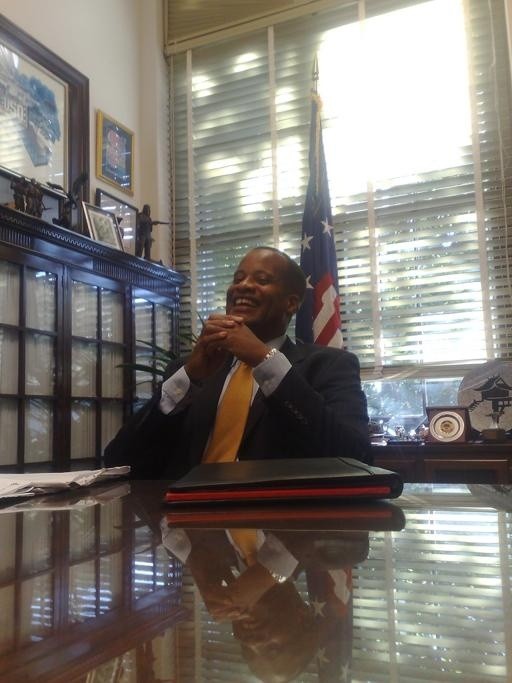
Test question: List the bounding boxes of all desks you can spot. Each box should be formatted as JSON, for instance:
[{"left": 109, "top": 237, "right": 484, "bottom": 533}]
[{"left": 0, "top": 467, "right": 512, "bottom": 681}]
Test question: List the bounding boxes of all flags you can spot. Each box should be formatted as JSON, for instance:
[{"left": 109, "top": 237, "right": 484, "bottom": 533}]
[
  {"left": 304, "top": 570, "right": 353, "bottom": 682},
  {"left": 297, "top": 97, "right": 345, "bottom": 351}
]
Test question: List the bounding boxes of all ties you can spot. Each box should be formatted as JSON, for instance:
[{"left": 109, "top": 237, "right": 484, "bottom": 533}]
[{"left": 202, "top": 358, "right": 255, "bottom": 463}]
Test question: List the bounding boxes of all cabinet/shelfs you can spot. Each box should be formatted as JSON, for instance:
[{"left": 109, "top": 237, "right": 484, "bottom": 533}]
[
  {"left": 422, "top": 435, "right": 512, "bottom": 486},
  {"left": 370, "top": 440, "right": 429, "bottom": 485},
  {"left": 1, "top": 200, "right": 183, "bottom": 475}
]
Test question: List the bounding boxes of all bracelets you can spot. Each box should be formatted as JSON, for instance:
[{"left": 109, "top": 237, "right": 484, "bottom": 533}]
[{"left": 263, "top": 346, "right": 279, "bottom": 363}]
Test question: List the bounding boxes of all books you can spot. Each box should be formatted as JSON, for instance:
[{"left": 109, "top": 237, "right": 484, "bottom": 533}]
[
  {"left": 164, "top": 457, "right": 403, "bottom": 505},
  {"left": 164, "top": 497, "right": 410, "bottom": 534}
]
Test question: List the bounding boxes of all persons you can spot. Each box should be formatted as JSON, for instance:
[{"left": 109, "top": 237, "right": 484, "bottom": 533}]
[
  {"left": 105, "top": 247, "right": 372, "bottom": 479},
  {"left": 122, "top": 491, "right": 368, "bottom": 683},
  {"left": 135, "top": 204, "right": 153, "bottom": 261},
  {"left": 10, "top": 176, "right": 43, "bottom": 216}
]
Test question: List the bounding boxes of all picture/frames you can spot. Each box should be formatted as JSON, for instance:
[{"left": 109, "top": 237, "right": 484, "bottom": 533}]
[
  {"left": 0, "top": 11, "right": 94, "bottom": 210},
  {"left": 77, "top": 197, "right": 124, "bottom": 250},
  {"left": 92, "top": 185, "right": 142, "bottom": 257},
  {"left": 97, "top": 105, "right": 140, "bottom": 197}
]
